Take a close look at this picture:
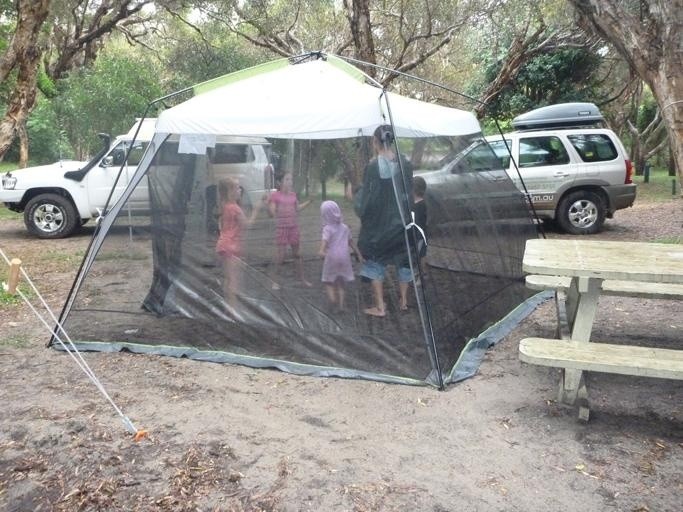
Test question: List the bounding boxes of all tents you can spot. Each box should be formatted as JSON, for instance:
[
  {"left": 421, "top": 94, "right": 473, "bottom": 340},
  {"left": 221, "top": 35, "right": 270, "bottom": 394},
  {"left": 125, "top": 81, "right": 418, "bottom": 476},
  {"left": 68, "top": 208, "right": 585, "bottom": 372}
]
[{"left": 47, "top": 50, "right": 560, "bottom": 393}]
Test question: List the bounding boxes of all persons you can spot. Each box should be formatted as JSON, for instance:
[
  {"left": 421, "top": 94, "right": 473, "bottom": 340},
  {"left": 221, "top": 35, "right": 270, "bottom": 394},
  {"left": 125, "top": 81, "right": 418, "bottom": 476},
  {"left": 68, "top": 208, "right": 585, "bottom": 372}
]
[
  {"left": 411, "top": 176, "right": 429, "bottom": 287},
  {"left": 357, "top": 125, "right": 419, "bottom": 318},
  {"left": 319, "top": 200, "right": 367, "bottom": 312},
  {"left": 267, "top": 168, "right": 312, "bottom": 290},
  {"left": 215, "top": 176, "right": 264, "bottom": 295}
]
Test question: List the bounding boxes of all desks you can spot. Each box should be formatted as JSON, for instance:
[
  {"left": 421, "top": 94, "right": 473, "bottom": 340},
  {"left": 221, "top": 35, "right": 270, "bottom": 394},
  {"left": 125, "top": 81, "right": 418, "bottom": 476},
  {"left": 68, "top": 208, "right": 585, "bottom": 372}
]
[{"left": 523, "top": 238, "right": 683, "bottom": 405}]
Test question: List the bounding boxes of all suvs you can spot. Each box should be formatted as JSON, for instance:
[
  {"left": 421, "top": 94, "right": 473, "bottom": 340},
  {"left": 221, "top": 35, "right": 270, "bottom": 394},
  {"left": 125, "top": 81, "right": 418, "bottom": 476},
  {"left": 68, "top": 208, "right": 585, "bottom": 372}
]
[
  {"left": 354, "top": 101, "right": 637, "bottom": 234},
  {"left": 0, "top": 118, "right": 278, "bottom": 239}
]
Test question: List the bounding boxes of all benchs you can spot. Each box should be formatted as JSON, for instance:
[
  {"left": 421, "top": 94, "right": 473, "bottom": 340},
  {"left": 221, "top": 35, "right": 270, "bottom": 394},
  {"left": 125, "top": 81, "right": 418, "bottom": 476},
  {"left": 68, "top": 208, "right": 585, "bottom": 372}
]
[{"left": 519, "top": 275, "right": 683, "bottom": 408}]
